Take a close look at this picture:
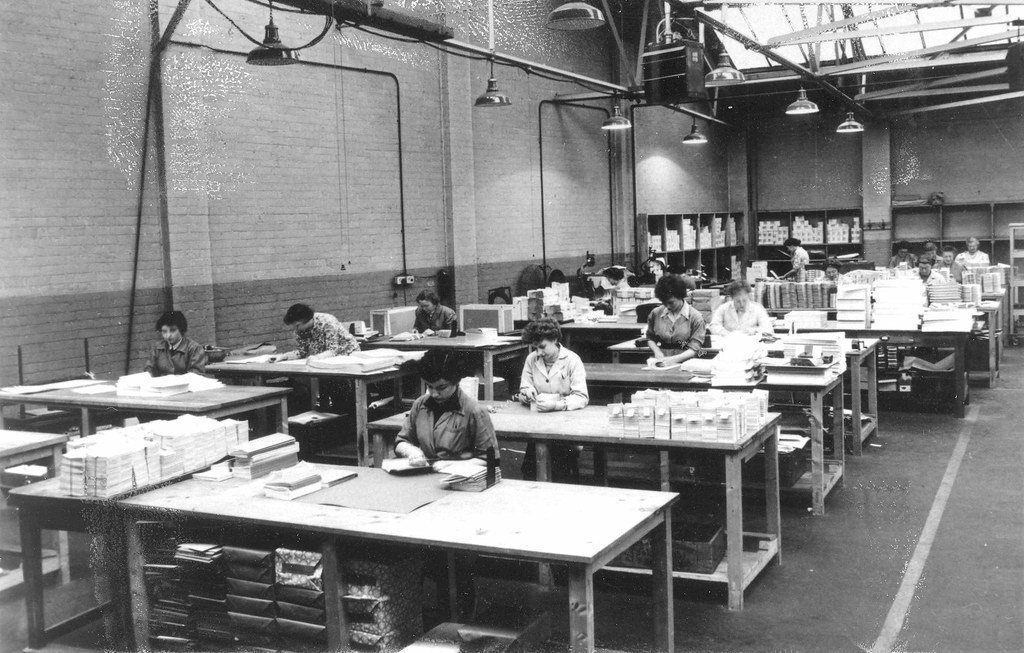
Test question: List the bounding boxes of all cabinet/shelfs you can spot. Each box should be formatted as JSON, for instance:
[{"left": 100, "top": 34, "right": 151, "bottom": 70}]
[{"left": 637, "top": 199, "right": 1024, "bottom": 327}]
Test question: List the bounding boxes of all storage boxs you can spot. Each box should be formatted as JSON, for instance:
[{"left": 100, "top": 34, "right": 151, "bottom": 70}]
[
  {"left": 459, "top": 304, "right": 513, "bottom": 332},
  {"left": 371, "top": 305, "right": 418, "bottom": 337}
]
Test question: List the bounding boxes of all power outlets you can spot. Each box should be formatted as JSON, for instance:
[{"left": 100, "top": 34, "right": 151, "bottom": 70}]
[{"left": 395, "top": 276, "right": 415, "bottom": 284}]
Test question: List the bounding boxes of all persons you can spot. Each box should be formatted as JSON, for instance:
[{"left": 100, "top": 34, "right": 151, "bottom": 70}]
[
  {"left": 818, "top": 258, "right": 850, "bottom": 282},
  {"left": 519, "top": 317, "right": 590, "bottom": 485},
  {"left": 779, "top": 237, "right": 810, "bottom": 280},
  {"left": 666, "top": 265, "right": 694, "bottom": 289},
  {"left": 646, "top": 275, "right": 706, "bottom": 367},
  {"left": 954, "top": 236, "right": 990, "bottom": 264},
  {"left": 888, "top": 240, "right": 967, "bottom": 286},
  {"left": 143, "top": 311, "right": 206, "bottom": 377},
  {"left": 414, "top": 288, "right": 457, "bottom": 337},
  {"left": 595, "top": 268, "right": 632, "bottom": 313},
  {"left": 266, "top": 303, "right": 364, "bottom": 415},
  {"left": 394, "top": 346, "right": 502, "bottom": 627},
  {"left": 707, "top": 282, "right": 777, "bottom": 345}
]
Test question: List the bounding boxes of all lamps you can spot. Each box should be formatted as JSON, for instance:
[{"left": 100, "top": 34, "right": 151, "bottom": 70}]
[
  {"left": 246, "top": 0, "right": 301, "bottom": 66},
  {"left": 682, "top": 116, "right": 708, "bottom": 144},
  {"left": 785, "top": 78, "right": 820, "bottom": 115},
  {"left": 836, "top": 104, "right": 865, "bottom": 133},
  {"left": 473, "top": 56, "right": 513, "bottom": 107},
  {"left": 601, "top": 88, "right": 633, "bottom": 129},
  {"left": 545, "top": 0, "right": 606, "bottom": 31},
  {"left": 703, "top": 32, "right": 746, "bottom": 85}
]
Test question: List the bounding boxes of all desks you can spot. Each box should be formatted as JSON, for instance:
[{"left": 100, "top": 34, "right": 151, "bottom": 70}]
[
  {"left": 6, "top": 462, "right": 681, "bottom": 653},
  {"left": 581, "top": 362, "right": 846, "bottom": 516},
  {"left": 365, "top": 398, "right": 784, "bottom": 614},
  {"left": 0, "top": 428, "right": 68, "bottom": 594},
  {"left": 774, "top": 318, "right": 976, "bottom": 418},
  {"left": 359, "top": 334, "right": 533, "bottom": 401},
  {"left": 204, "top": 356, "right": 424, "bottom": 468},
  {"left": 560, "top": 321, "right": 649, "bottom": 350},
  {"left": 927, "top": 301, "right": 1002, "bottom": 388},
  {"left": 607, "top": 337, "right": 882, "bottom": 456},
  {"left": 981, "top": 289, "right": 1007, "bottom": 314},
  {"left": 0, "top": 379, "right": 293, "bottom": 511}
]
